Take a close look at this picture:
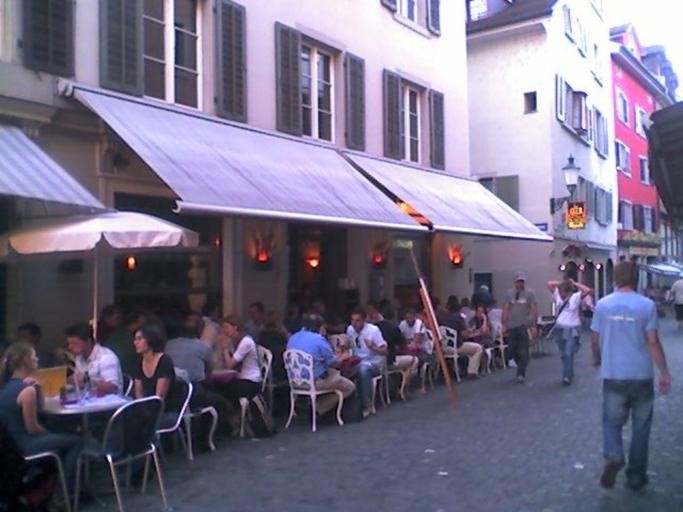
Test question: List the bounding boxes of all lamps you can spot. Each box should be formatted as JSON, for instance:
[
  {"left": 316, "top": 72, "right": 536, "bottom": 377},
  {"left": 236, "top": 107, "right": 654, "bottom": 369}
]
[{"left": 551, "top": 154, "right": 580, "bottom": 214}]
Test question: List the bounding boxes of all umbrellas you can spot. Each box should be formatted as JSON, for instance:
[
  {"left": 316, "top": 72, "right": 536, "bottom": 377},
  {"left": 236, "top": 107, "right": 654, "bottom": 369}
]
[{"left": 0, "top": 208, "right": 201, "bottom": 344}]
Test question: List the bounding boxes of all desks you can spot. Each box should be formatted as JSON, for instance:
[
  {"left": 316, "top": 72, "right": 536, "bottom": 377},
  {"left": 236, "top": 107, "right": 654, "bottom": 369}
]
[{"left": 35, "top": 391, "right": 137, "bottom": 509}]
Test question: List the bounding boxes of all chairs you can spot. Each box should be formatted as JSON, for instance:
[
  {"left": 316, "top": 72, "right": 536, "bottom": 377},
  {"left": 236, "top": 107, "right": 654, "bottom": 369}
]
[
  {"left": 303, "top": 320, "right": 555, "bottom": 415},
  {"left": 162, "top": 343, "right": 274, "bottom": 463},
  {"left": 80, "top": 394, "right": 170, "bottom": 511},
  {"left": 1, "top": 419, "right": 72, "bottom": 511},
  {"left": 122, "top": 378, "right": 193, "bottom": 489},
  {"left": 283, "top": 348, "right": 347, "bottom": 433},
  {"left": 118, "top": 371, "right": 134, "bottom": 397}
]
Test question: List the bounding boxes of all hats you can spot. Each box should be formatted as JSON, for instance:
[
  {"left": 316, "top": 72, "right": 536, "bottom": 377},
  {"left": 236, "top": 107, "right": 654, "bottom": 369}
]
[{"left": 514, "top": 275, "right": 525, "bottom": 282}]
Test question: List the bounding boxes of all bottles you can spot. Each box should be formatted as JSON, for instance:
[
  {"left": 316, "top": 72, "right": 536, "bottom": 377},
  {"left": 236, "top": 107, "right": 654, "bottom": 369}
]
[{"left": 83, "top": 370, "right": 92, "bottom": 400}]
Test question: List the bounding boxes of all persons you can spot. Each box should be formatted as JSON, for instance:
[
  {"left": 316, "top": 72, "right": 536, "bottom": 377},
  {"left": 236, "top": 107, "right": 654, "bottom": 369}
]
[
  {"left": 1, "top": 304, "right": 181, "bottom": 490},
  {"left": 164, "top": 286, "right": 502, "bottom": 438},
  {"left": 500, "top": 271, "right": 539, "bottom": 385},
  {"left": 666, "top": 271, "right": 682, "bottom": 328},
  {"left": 545, "top": 276, "right": 593, "bottom": 384},
  {"left": 584, "top": 260, "right": 673, "bottom": 491}
]
